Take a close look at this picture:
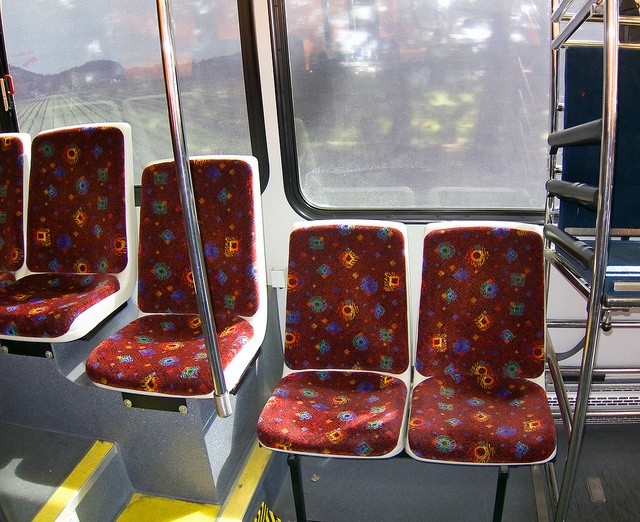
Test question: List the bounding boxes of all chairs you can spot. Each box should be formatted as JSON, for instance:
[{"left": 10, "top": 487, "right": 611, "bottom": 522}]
[
  {"left": 558, "top": 45, "right": 639, "bottom": 297},
  {"left": 258, "top": 220, "right": 410, "bottom": 460},
  {"left": 1, "top": 133, "right": 32, "bottom": 288},
  {"left": 86, "top": 154, "right": 267, "bottom": 395},
  {"left": 1, "top": 122, "right": 137, "bottom": 342},
  {"left": 408, "top": 221, "right": 557, "bottom": 463}
]
